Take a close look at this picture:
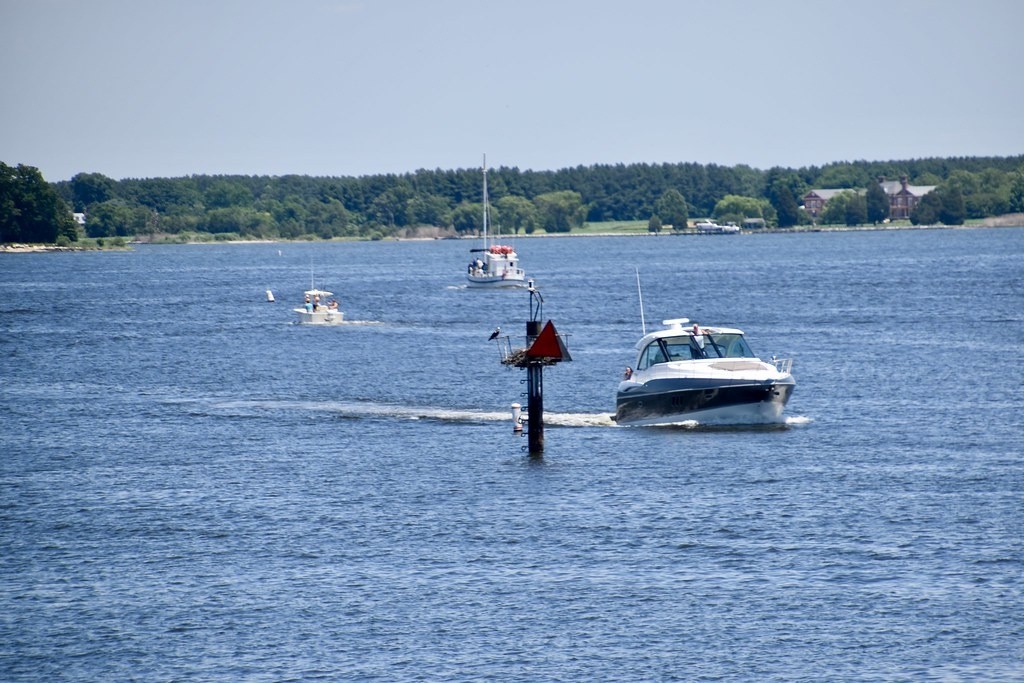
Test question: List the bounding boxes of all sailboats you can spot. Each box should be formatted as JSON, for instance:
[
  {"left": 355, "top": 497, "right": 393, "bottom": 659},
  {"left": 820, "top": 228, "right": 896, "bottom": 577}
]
[{"left": 466, "top": 152, "right": 525, "bottom": 288}]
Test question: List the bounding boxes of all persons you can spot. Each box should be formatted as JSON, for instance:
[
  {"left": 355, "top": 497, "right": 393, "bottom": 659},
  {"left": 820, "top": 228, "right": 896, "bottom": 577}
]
[
  {"left": 623, "top": 366, "right": 633, "bottom": 380},
  {"left": 301, "top": 296, "right": 338, "bottom": 313},
  {"left": 689, "top": 324, "right": 711, "bottom": 359},
  {"left": 656, "top": 340, "right": 671, "bottom": 356},
  {"left": 476, "top": 257, "right": 484, "bottom": 269}
]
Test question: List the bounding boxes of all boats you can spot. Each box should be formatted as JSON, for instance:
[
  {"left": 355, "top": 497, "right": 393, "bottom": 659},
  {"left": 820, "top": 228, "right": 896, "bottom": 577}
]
[
  {"left": 611, "top": 265, "right": 797, "bottom": 422},
  {"left": 293, "top": 254, "right": 343, "bottom": 323},
  {"left": 696, "top": 219, "right": 740, "bottom": 233}
]
